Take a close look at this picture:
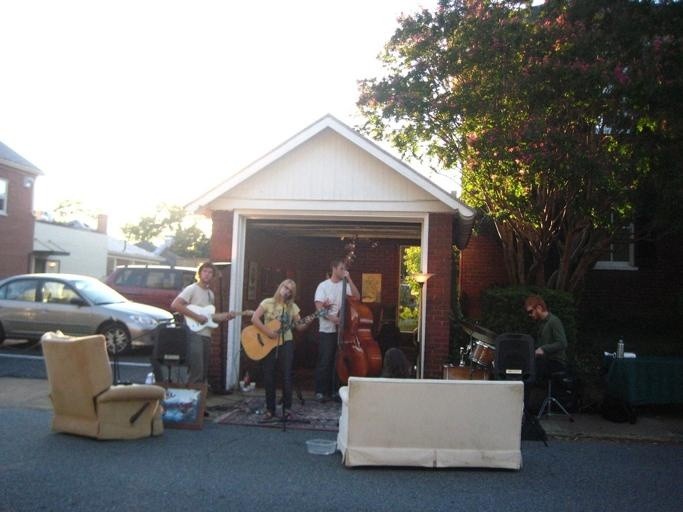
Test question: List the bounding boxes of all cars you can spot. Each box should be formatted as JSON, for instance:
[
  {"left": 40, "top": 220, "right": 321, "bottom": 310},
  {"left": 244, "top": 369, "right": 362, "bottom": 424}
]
[{"left": 0, "top": 271, "right": 175, "bottom": 359}]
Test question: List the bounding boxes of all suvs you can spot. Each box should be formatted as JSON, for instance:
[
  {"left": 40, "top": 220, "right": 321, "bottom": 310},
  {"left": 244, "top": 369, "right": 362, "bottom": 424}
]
[{"left": 105, "top": 262, "right": 198, "bottom": 325}]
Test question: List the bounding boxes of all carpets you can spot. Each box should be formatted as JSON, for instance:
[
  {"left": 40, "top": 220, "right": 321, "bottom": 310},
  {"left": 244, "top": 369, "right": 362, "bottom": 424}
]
[{"left": 212, "top": 395, "right": 341, "bottom": 432}]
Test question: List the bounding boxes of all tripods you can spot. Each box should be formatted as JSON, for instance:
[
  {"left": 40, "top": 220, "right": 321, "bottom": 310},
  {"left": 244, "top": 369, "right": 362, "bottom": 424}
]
[{"left": 258, "top": 304, "right": 311, "bottom": 431}]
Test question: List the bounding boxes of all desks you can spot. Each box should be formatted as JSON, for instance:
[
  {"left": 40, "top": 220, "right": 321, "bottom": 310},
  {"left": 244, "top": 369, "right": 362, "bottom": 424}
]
[{"left": 608, "top": 356, "right": 682, "bottom": 424}]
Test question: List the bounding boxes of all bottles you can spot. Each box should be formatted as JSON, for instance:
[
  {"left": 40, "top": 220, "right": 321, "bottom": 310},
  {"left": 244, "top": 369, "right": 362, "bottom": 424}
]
[{"left": 617, "top": 339, "right": 625, "bottom": 359}]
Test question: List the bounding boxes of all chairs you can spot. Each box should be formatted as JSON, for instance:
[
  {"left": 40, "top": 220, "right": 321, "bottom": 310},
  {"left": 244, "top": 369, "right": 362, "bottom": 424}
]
[
  {"left": 40, "top": 331, "right": 166, "bottom": 440},
  {"left": 534, "top": 340, "right": 576, "bottom": 423}
]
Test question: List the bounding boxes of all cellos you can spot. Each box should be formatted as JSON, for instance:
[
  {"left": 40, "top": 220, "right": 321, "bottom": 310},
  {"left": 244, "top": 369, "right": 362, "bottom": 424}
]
[{"left": 335, "top": 234, "right": 382, "bottom": 382}]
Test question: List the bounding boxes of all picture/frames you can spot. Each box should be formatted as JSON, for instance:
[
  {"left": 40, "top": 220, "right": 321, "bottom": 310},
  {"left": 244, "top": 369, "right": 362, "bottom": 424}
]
[{"left": 153, "top": 382, "right": 208, "bottom": 429}]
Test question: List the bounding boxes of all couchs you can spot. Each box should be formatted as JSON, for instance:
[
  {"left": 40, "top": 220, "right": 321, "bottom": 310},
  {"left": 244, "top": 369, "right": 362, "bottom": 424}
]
[{"left": 336, "top": 376, "right": 524, "bottom": 469}]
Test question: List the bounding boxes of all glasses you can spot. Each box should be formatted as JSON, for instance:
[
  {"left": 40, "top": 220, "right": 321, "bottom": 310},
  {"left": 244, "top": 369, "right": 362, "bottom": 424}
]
[{"left": 527, "top": 309, "right": 534, "bottom": 315}]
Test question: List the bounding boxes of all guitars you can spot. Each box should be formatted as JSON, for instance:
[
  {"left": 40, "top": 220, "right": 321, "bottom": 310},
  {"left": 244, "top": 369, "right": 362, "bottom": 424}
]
[{"left": 240, "top": 302, "right": 336, "bottom": 361}]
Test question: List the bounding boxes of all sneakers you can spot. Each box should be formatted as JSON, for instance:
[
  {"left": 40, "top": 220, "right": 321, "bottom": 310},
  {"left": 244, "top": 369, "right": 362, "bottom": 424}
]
[
  {"left": 263, "top": 410, "right": 272, "bottom": 420},
  {"left": 314, "top": 392, "right": 324, "bottom": 402},
  {"left": 330, "top": 393, "right": 341, "bottom": 402}
]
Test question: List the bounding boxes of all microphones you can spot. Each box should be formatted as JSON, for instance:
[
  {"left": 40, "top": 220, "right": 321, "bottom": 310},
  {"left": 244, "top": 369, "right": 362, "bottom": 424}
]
[{"left": 285, "top": 292, "right": 293, "bottom": 299}]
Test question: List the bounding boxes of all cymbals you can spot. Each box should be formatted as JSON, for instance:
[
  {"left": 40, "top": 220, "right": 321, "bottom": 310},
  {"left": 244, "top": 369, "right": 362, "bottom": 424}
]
[{"left": 462, "top": 324, "right": 496, "bottom": 345}]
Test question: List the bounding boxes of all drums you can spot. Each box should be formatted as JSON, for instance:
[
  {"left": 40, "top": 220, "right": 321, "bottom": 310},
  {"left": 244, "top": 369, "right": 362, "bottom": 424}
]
[
  {"left": 469, "top": 340, "right": 496, "bottom": 367},
  {"left": 442, "top": 365, "right": 493, "bottom": 380}
]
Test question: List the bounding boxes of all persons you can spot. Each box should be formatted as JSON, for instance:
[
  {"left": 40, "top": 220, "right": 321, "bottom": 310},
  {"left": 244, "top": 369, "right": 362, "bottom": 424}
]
[
  {"left": 312, "top": 259, "right": 361, "bottom": 402},
  {"left": 523, "top": 292, "right": 569, "bottom": 379},
  {"left": 250, "top": 278, "right": 315, "bottom": 420},
  {"left": 170, "top": 260, "right": 237, "bottom": 418}
]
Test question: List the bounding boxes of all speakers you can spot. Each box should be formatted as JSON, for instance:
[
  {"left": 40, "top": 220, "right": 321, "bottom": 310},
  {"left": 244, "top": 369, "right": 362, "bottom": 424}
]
[
  {"left": 153, "top": 326, "right": 188, "bottom": 362},
  {"left": 495, "top": 333, "right": 536, "bottom": 388}
]
